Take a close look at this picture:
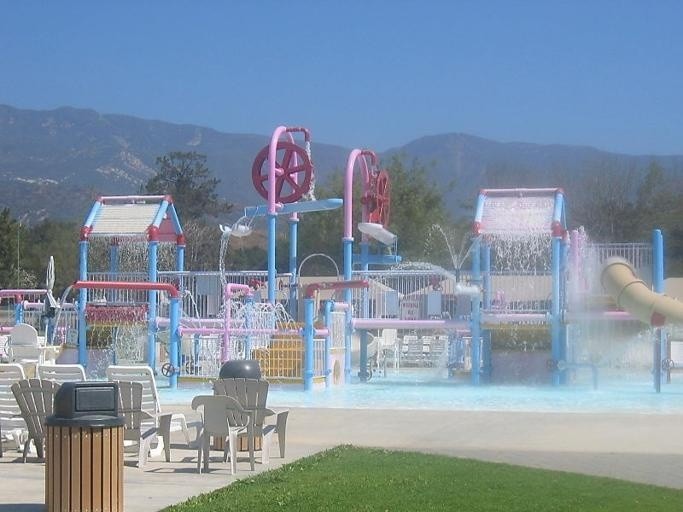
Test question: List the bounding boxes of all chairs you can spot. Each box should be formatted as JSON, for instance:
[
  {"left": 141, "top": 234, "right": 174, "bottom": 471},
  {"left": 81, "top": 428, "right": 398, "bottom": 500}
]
[
  {"left": 0, "top": 364, "right": 289, "bottom": 476},
  {"left": 378, "top": 329, "right": 448, "bottom": 368},
  {"left": 8, "top": 324, "right": 42, "bottom": 363}
]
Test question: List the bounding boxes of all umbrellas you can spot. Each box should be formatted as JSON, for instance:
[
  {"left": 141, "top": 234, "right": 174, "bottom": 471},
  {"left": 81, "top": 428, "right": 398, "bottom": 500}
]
[{"left": 43, "top": 254, "right": 62, "bottom": 347}]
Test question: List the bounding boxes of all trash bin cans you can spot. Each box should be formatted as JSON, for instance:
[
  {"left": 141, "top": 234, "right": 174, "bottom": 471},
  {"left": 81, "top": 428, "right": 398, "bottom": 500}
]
[
  {"left": 213, "top": 360, "right": 262, "bottom": 450},
  {"left": 43, "top": 381, "right": 123, "bottom": 512}
]
